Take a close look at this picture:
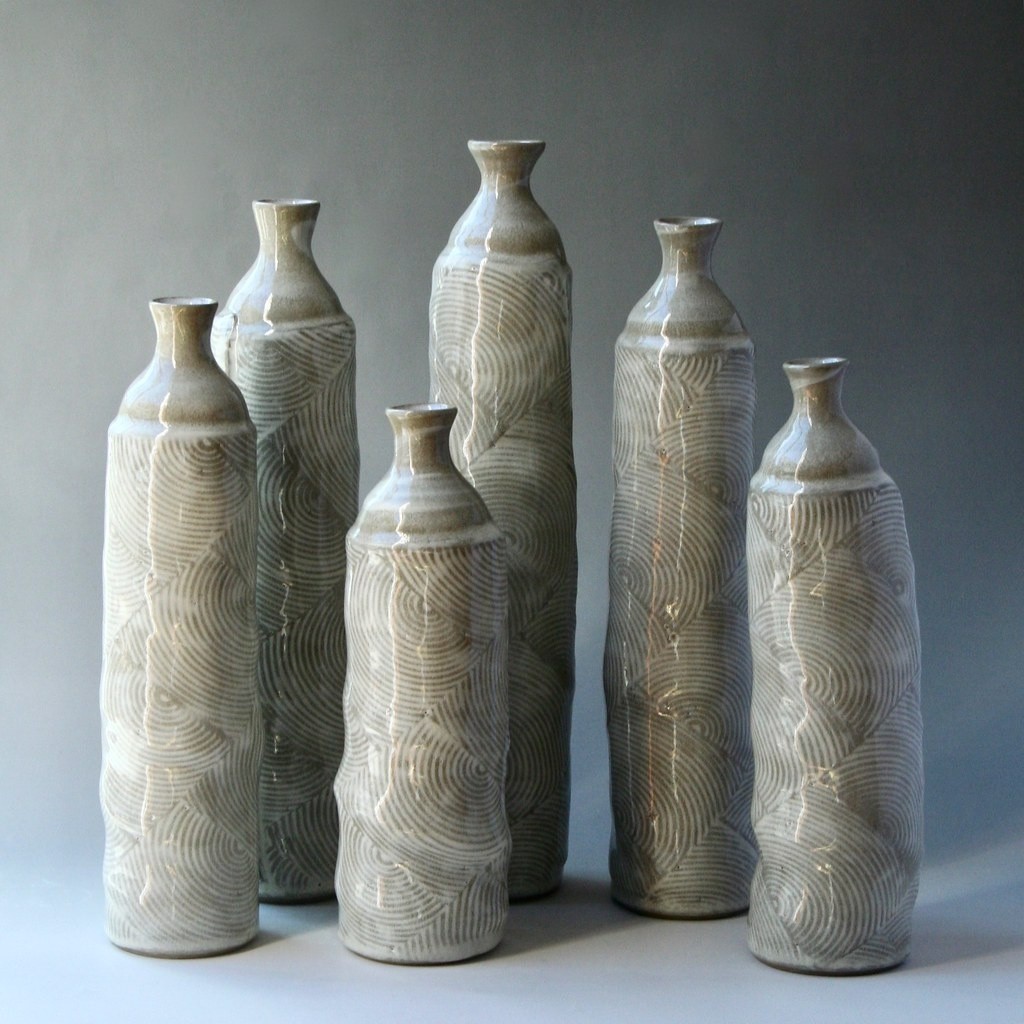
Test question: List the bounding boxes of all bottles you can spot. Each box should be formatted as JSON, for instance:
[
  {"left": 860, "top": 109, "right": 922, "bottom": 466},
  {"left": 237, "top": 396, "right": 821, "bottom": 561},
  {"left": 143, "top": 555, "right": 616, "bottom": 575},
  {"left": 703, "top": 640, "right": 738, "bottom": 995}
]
[
  {"left": 333, "top": 402, "right": 514, "bottom": 967},
  {"left": 427, "top": 134, "right": 577, "bottom": 904},
  {"left": 744, "top": 357, "right": 924, "bottom": 976},
  {"left": 99, "top": 295, "right": 263, "bottom": 961},
  {"left": 603, "top": 213, "right": 762, "bottom": 924},
  {"left": 212, "top": 196, "right": 361, "bottom": 905}
]
